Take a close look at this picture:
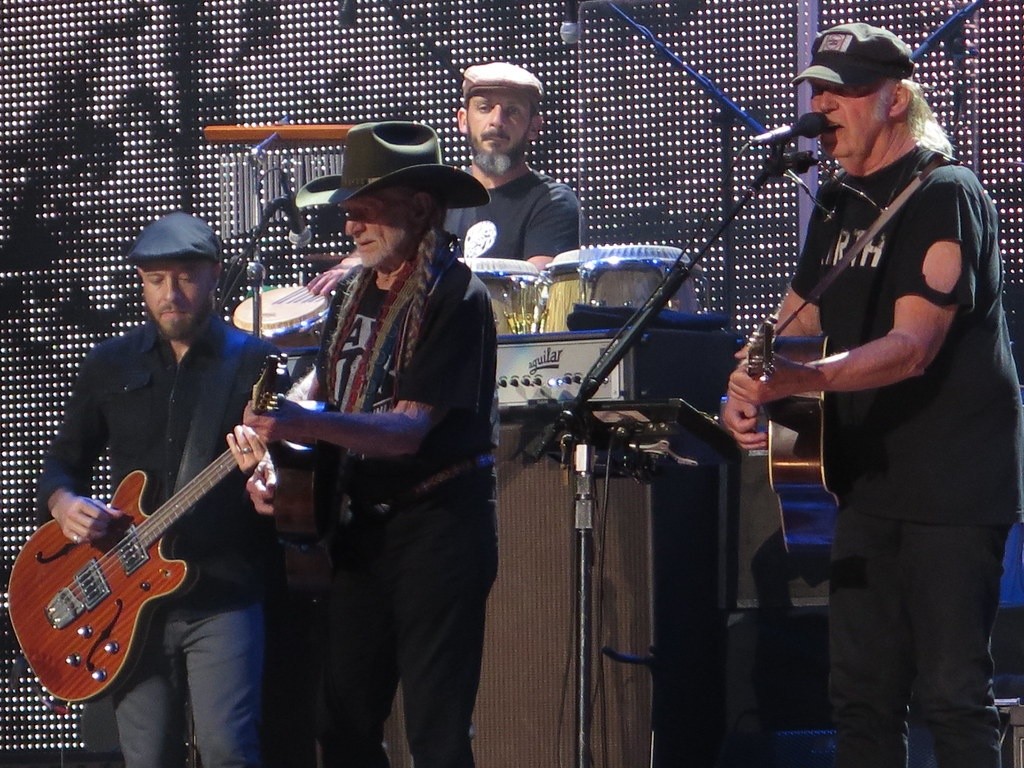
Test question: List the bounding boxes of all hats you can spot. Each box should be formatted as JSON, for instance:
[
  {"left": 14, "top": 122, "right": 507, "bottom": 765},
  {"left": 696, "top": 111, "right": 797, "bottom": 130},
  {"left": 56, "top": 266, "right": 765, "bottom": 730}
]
[
  {"left": 127, "top": 211, "right": 222, "bottom": 264},
  {"left": 295, "top": 121, "right": 489, "bottom": 207},
  {"left": 791, "top": 23, "right": 914, "bottom": 85},
  {"left": 462, "top": 62, "right": 544, "bottom": 107}
]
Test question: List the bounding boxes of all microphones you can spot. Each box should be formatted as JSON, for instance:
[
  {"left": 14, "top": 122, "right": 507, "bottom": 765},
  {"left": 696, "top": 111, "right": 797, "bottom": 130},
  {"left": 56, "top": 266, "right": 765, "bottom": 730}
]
[
  {"left": 280, "top": 171, "right": 312, "bottom": 247},
  {"left": 749, "top": 112, "right": 828, "bottom": 146},
  {"left": 560, "top": 0, "right": 580, "bottom": 44}
]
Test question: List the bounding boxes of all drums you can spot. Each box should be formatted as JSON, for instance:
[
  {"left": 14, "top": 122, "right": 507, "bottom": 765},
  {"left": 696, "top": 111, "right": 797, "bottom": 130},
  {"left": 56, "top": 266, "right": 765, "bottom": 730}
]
[
  {"left": 454, "top": 255, "right": 540, "bottom": 336},
  {"left": 542, "top": 242, "right": 697, "bottom": 332},
  {"left": 230, "top": 284, "right": 332, "bottom": 347}
]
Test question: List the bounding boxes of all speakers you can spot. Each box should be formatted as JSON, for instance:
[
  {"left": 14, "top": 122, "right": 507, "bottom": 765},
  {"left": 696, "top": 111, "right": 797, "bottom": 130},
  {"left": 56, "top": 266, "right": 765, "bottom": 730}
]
[{"left": 314, "top": 409, "right": 1013, "bottom": 768}]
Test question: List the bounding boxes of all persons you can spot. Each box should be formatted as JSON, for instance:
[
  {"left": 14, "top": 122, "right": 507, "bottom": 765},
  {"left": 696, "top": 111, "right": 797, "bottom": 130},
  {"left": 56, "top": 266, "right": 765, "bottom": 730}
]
[
  {"left": 37, "top": 212, "right": 293, "bottom": 768},
  {"left": 244, "top": 121, "right": 499, "bottom": 768},
  {"left": 306, "top": 62, "right": 582, "bottom": 297},
  {"left": 725, "top": 24, "right": 1024, "bottom": 768}
]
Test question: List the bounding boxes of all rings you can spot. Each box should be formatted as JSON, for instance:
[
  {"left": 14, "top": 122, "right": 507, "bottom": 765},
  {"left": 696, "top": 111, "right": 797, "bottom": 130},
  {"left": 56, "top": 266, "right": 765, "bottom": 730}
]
[
  {"left": 242, "top": 448, "right": 252, "bottom": 454},
  {"left": 73, "top": 535, "right": 79, "bottom": 542}
]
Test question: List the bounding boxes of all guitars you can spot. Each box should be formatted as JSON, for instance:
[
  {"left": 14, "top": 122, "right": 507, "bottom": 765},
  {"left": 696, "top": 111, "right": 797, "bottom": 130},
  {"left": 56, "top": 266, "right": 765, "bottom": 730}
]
[
  {"left": 6, "top": 360, "right": 315, "bottom": 706},
  {"left": 744, "top": 317, "right": 843, "bottom": 557},
  {"left": 252, "top": 350, "right": 326, "bottom": 553}
]
